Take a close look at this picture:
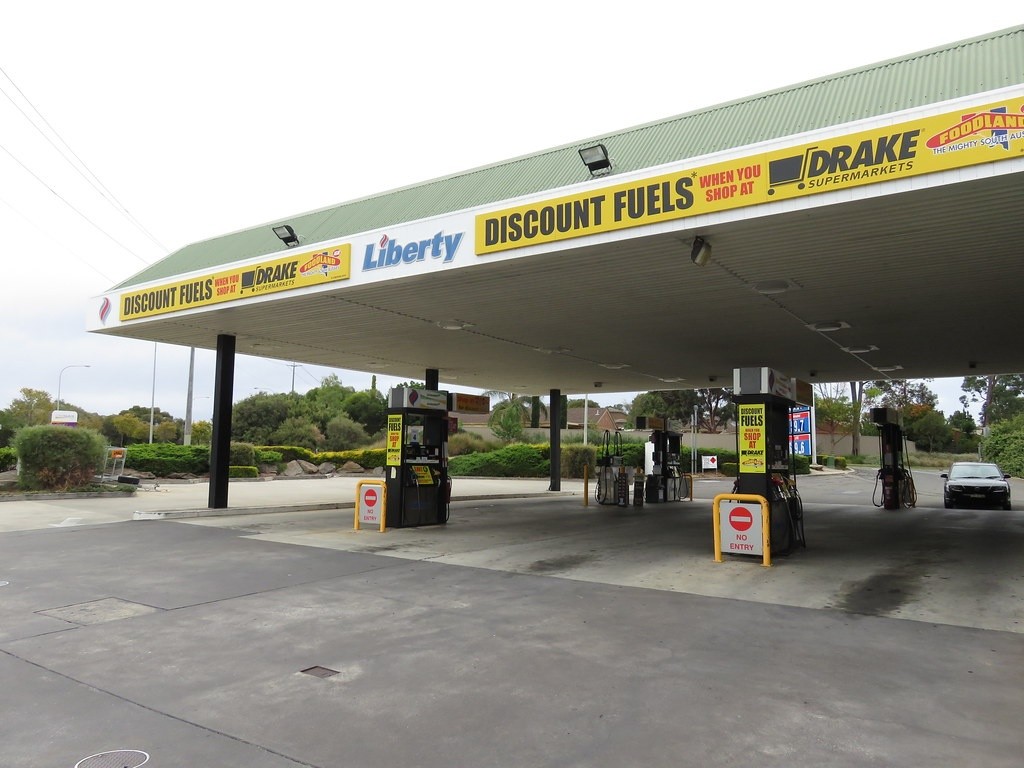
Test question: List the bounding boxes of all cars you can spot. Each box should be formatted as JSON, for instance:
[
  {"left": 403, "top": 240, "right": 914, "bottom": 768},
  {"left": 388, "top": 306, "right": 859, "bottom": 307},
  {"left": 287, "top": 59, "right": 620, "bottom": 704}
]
[{"left": 940, "top": 462, "right": 1012, "bottom": 511}]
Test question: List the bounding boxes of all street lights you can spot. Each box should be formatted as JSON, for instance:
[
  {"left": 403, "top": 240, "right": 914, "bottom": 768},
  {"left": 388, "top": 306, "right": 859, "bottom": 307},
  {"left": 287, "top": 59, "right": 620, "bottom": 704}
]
[{"left": 57, "top": 364, "right": 91, "bottom": 410}]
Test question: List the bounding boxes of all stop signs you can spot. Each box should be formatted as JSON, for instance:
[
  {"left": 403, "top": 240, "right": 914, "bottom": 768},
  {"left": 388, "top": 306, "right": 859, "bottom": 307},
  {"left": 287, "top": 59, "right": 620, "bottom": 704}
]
[
  {"left": 728, "top": 506, "right": 753, "bottom": 532},
  {"left": 364, "top": 489, "right": 377, "bottom": 506}
]
